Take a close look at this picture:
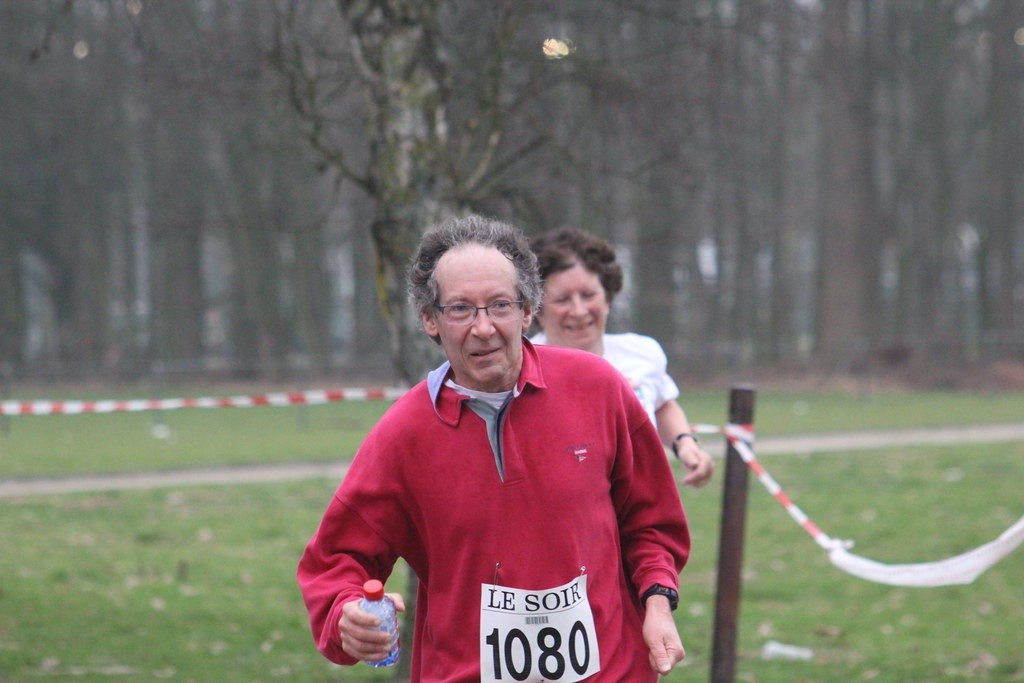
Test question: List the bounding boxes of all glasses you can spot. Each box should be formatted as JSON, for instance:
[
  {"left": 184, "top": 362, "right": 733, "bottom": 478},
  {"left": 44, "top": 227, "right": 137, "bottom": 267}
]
[{"left": 434, "top": 296, "right": 525, "bottom": 326}]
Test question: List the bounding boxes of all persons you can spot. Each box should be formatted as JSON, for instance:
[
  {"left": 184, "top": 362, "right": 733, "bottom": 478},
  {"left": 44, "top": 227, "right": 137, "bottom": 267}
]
[{"left": 296, "top": 216, "right": 714, "bottom": 683}]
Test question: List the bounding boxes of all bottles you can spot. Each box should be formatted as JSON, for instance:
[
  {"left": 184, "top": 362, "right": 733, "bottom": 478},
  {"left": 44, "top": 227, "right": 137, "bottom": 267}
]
[{"left": 358, "top": 580, "right": 401, "bottom": 666}]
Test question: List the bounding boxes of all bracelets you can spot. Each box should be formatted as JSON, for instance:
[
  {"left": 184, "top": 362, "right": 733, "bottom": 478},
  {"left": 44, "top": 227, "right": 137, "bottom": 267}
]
[
  {"left": 641, "top": 584, "right": 686, "bottom": 611},
  {"left": 670, "top": 432, "right": 697, "bottom": 457}
]
